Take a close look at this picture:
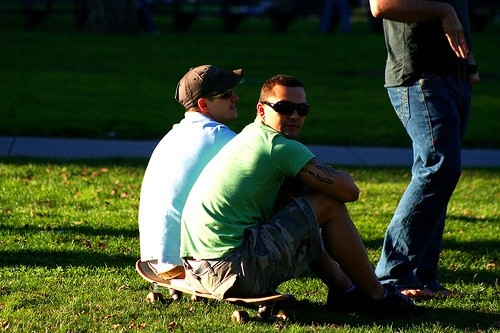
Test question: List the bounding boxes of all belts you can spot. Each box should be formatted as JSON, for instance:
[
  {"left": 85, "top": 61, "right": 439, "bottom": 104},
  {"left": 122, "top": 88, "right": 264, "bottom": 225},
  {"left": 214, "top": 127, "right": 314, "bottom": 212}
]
[{"left": 146, "top": 262, "right": 184, "bottom": 280}]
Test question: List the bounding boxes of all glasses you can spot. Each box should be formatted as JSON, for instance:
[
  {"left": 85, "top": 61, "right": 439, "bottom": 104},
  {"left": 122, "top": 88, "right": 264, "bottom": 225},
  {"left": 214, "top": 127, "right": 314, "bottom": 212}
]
[
  {"left": 208, "top": 89, "right": 233, "bottom": 98},
  {"left": 262, "top": 100, "right": 310, "bottom": 115}
]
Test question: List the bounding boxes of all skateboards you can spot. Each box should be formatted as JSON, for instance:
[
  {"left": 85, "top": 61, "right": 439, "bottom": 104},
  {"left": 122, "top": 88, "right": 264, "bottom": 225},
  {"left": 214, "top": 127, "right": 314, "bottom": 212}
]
[{"left": 135, "top": 258, "right": 295, "bottom": 325}]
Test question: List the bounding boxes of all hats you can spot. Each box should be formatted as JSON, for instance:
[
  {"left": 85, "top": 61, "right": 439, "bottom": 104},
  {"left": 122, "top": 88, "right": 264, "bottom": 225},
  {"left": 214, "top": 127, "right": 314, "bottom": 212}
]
[{"left": 175, "top": 64, "right": 243, "bottom": 108}]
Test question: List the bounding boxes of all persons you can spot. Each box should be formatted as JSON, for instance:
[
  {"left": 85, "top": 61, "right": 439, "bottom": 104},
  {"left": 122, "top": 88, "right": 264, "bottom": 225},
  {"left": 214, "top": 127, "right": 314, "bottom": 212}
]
[
  {"left": 370, "top": 0, "right": 480, "bottom": 302},
  {"left": 180, "top": 75, "right": 419, "bottom": 319},
  {"left": 138, "top": 65, "right": 243, "bottom": 283}
]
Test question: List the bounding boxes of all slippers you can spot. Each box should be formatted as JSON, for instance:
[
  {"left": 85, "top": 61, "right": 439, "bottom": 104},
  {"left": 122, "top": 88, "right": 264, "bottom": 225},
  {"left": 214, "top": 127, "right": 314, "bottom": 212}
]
[{"left": 393, "top": 280, "right": 455, "bottom": 300}]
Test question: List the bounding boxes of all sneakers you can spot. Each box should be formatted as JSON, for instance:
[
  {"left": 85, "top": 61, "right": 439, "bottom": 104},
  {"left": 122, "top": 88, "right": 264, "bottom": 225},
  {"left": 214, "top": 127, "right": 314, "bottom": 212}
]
[{"left": 327, "top": 283, "right": 427, "bottom": 319}]
